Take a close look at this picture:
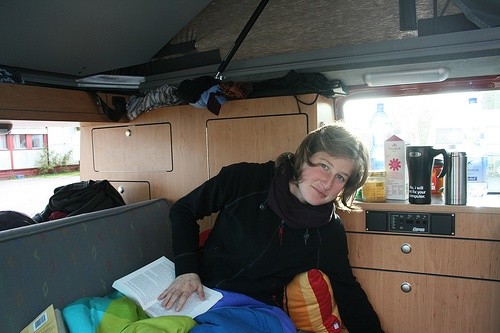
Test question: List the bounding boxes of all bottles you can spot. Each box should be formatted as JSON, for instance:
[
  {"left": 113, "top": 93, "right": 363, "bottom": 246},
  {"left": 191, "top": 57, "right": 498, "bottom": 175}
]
[{"left": 467, "top": 143, "right": 488, "bottom": 198}]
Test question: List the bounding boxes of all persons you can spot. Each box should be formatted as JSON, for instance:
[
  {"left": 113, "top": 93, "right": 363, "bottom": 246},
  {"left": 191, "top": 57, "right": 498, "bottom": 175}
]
[{"left": 158, "top": 125, "right": 387, "bottom": 333}]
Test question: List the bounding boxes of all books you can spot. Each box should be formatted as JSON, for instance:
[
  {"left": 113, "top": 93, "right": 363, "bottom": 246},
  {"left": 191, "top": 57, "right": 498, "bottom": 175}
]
[
  {"left": 19, "top": 304, "right": 68, "bottom": 333},
  {"left": 112, "top": 255, "right": 223, "bottom": 324}
]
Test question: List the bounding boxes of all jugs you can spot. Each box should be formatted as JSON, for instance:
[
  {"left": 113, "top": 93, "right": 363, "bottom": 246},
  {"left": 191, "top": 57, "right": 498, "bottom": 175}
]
[{"left": 406, "top": 146, "right": 448, "bottom": 205}]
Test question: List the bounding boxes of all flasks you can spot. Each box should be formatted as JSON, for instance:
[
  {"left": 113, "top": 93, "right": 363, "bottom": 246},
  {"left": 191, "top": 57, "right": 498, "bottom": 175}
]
[{"left": 445, "top": 152, "right": 467, "bottom": 205}]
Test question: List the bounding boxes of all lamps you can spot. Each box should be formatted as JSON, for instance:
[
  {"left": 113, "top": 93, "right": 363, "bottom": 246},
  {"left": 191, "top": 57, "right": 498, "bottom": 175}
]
[{"left": 363, "top": 66, "right": 452, "bottom": 88}]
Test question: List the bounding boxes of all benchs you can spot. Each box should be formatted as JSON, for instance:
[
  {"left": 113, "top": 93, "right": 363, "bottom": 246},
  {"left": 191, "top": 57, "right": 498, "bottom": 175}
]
[{"left": 0, "top": 198, "right": 176, "bottom": 333}]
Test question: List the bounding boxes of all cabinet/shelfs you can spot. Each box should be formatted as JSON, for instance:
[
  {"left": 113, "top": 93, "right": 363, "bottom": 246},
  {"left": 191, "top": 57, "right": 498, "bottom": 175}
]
[
  {"left": 334, "top": 197, "right": 500, "bottom": 333},
  {"left": 79, "top": 93, "right": 336, "bottom": 207}
]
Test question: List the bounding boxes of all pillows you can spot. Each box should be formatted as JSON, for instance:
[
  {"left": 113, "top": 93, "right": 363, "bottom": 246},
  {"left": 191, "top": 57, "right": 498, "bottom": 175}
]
[{"left": 281, "top": 268, "right": 348, "bottom": 333}]
